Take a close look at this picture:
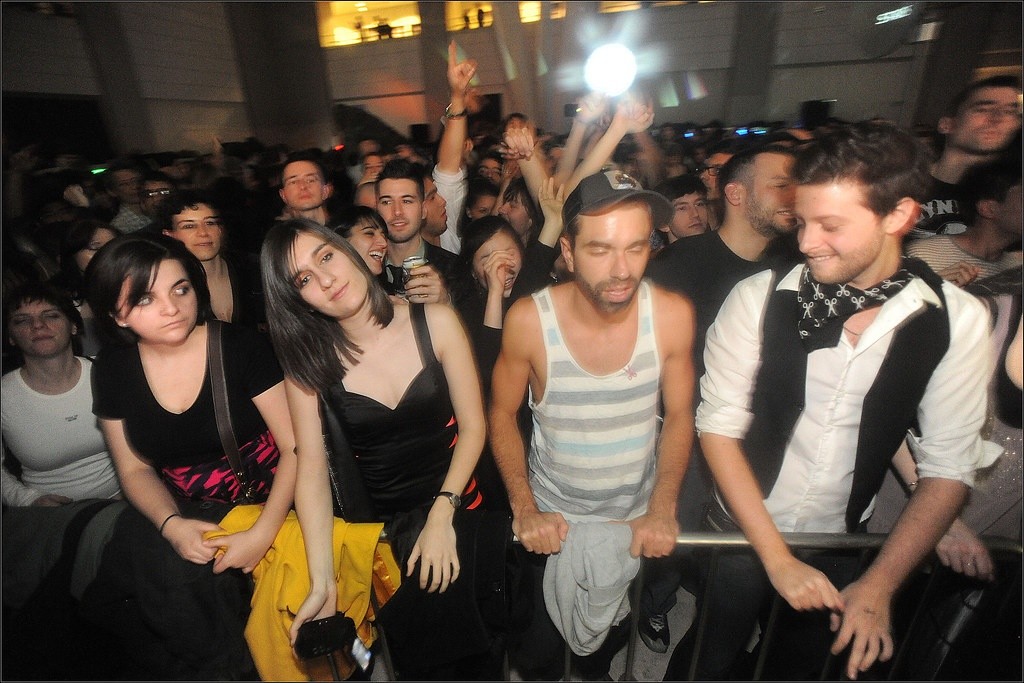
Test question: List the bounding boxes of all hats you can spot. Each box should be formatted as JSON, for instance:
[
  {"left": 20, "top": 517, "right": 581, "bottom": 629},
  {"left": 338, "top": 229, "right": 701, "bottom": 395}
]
[
  {"left": 940, "top": 64, "right": 1022, "bottom": 107},
  {"left": 561, "top": 170, "right": 675, "bottom": 229}
]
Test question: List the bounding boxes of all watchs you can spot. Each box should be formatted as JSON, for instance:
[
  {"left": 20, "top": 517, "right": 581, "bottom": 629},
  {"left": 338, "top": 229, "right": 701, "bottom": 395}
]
[{"left": 434, "top": 491, "right": 461, "bottom": 510}]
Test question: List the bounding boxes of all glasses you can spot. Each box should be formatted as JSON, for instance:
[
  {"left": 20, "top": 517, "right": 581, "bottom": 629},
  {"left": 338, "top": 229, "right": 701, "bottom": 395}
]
[
  {"left": 695, "top": 163, "right": 722, "bottom": 177},
  {"left": 114, "top": 178, "right": 142, "bottom": 188},
  {"left": 141, "top": 188, "right": 175, "bottom": 198}
]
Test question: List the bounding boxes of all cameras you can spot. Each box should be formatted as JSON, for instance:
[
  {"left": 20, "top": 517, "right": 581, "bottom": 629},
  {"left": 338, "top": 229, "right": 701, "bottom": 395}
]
[{"left": 294, "top": 615, "right": 375, "bottom": 678}]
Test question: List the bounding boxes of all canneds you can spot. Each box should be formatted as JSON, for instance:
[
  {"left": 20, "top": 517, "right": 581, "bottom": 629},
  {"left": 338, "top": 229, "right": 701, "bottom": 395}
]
[{"left": 402, "top": 256, "right": 428, "bottom": 299}]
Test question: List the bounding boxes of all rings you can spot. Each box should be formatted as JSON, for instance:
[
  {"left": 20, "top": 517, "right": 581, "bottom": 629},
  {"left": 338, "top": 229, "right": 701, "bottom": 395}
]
[{"left": 962, "top": 563, "right": 975, "bottom": 566}]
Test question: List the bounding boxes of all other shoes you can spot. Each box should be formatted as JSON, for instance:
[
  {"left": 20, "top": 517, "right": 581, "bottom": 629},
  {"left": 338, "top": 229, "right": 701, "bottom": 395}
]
[{"left": 639, "top": 615, "right": 670, "bottom": 653}]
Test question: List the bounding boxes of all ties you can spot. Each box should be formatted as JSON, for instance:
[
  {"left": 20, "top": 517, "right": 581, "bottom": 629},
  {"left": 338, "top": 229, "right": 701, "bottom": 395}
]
[{"left": 387, "top": 264, "right": 404, "bottom": 291}]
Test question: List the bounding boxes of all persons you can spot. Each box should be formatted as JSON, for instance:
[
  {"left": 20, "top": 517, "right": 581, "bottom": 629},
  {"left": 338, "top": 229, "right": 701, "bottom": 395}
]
[
  {"left": 2, "top": 40, "right": 1023, "bottom": 650},
  {"left": 665, "top": 123, "right": 1005, "bottom": 682},
  {"left": 489, "top": 170, "right": 695, "bottom": 682},
  {"left": 2, "top": 288, "right": 126, "bottom": 508},
  {"left": 840, "top": 291, "right": 1022, "bottom": 681},
  {"left": 84, "top": 236, "right": 297, "bottom": 574},
  {"left": 261, "top": 218, "right": 505, "bottom": 681}
]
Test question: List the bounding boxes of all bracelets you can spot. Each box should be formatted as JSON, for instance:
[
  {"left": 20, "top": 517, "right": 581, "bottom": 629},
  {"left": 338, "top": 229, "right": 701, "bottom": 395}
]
[
  {"left": 907, "top": 481, "right": 918, "bottom": 488},
  {"left": 444, "top": 103, "right": 468, "bottom": 119},
  {"left": 160, "top": 514, "right": 181, "bottom": 538}
]
